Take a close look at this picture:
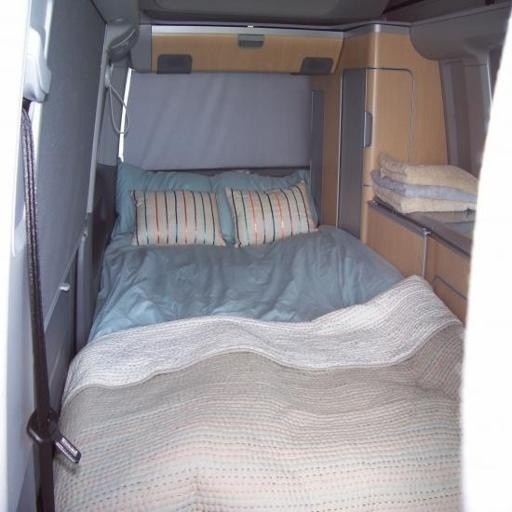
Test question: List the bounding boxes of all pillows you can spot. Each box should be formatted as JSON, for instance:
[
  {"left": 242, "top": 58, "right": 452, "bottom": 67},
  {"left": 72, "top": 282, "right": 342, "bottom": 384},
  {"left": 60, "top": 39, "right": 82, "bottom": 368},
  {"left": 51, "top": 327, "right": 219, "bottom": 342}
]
[
  {"left": 111, "top": 158, "right": 212, "bottom": 243},
  {"left": 226, "top": 180, "right": 319, "bottom": 247},
  {"left": 211, "top": 168, "right": 318, "bottom": 239},
  {"left": 129, "top": 189, "right": 227, "bottom": 247}
]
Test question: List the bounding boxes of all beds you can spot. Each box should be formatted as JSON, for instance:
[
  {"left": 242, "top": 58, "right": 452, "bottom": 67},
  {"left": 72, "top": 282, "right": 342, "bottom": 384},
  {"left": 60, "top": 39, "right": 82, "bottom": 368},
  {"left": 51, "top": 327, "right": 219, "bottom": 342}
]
[{"left": 52, "top": 157, "right": 461, "bottom": 512}]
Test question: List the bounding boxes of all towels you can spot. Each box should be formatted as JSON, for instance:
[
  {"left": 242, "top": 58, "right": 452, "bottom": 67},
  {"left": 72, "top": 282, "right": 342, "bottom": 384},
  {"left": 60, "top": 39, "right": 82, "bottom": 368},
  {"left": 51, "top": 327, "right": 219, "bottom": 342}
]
[{"left": 368, "top": 154, "right": 477, "bottom": 212}]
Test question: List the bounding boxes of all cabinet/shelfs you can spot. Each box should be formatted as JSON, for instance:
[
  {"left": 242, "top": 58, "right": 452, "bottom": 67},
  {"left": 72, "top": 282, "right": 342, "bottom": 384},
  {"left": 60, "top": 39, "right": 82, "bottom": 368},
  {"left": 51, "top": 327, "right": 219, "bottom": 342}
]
[{"left": 367, "top": 195, "right": 476, "bottom": 326}]
